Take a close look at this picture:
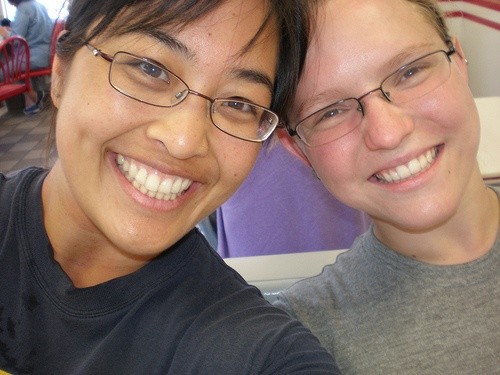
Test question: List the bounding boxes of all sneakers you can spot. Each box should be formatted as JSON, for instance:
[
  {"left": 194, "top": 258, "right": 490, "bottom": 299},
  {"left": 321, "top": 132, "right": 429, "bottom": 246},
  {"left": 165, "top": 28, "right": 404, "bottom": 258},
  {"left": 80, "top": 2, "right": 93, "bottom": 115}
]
[{"left": 23, "top": 90, "right": 45, "bottom": 113}]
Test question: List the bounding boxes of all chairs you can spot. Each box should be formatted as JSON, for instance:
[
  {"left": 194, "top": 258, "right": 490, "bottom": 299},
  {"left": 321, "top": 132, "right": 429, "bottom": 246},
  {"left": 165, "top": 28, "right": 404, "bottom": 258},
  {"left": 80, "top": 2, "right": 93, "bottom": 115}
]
[
  {"left": 21, "top": 20, "right": 65, "bottom": 80},
  {"left": 0, "top": 35, "right": 31, "bottom": 102}
]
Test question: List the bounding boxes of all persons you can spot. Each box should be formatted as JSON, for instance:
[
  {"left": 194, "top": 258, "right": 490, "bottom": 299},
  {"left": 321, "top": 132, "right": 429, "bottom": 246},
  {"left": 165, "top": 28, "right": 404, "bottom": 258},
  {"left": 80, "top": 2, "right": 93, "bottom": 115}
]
[
  {"left": 1, "top": 1, "right": 339, "bottom": 375},
  {"left": 1, "top": 0, "right": 52, "bottom": 115},
  {"left": 263, "top": 2, "right": 499, "bottom": 375}
]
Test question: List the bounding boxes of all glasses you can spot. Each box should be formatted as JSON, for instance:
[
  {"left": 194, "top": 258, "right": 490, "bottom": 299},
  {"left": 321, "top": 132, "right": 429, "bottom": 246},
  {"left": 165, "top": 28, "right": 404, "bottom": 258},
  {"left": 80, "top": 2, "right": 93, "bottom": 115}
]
[
  {"left": 283, "top": 40, "right": 456, "bottom": 147},
  {"left": 78, "top": 36, "right": 284, "bottom": 144}
]
